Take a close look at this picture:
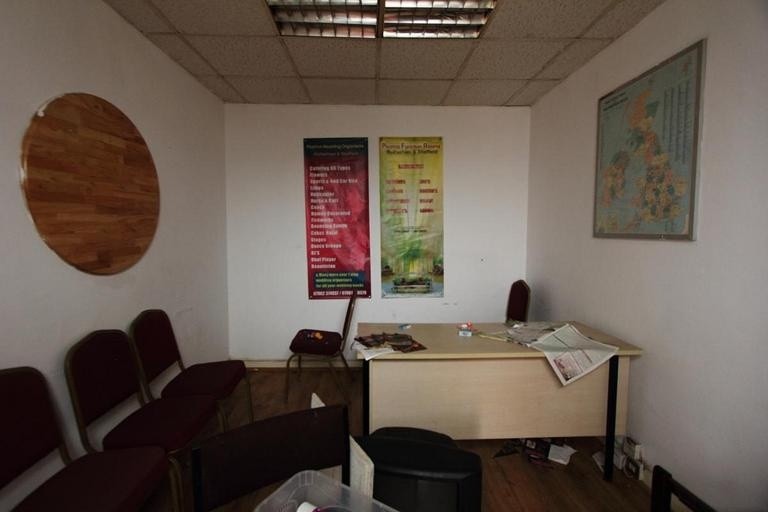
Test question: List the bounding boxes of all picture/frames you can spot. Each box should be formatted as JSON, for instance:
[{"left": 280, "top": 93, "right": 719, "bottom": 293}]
[{"left": 591, "top": 36, "right": 710, "bottom": 244}]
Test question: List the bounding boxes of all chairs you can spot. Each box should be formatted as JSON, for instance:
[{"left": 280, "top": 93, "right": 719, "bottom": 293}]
[
  {"left": 499, "top": 279, "right": 530, "bottom": 323},
  {"left": 287, "top": 287, "right": 357, "bottom": 403},
  {"left": 166, "top": 403, "right": 350, "bottom": 511},
  {"left": 345, "top": 427, "right": 481, "bottom": 511}
]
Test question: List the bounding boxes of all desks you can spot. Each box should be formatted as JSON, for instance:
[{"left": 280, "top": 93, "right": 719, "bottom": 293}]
[{"left": 354, "top": 323, "right": 647, "bottom": 485}]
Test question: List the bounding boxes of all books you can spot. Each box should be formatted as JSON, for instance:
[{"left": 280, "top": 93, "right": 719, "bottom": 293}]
[
  {"left": 521, "top": 438, "right": 577, "bottom": 470},
  {"left": 350, "top": 331, "right": 427, "bottom": 362}
]
